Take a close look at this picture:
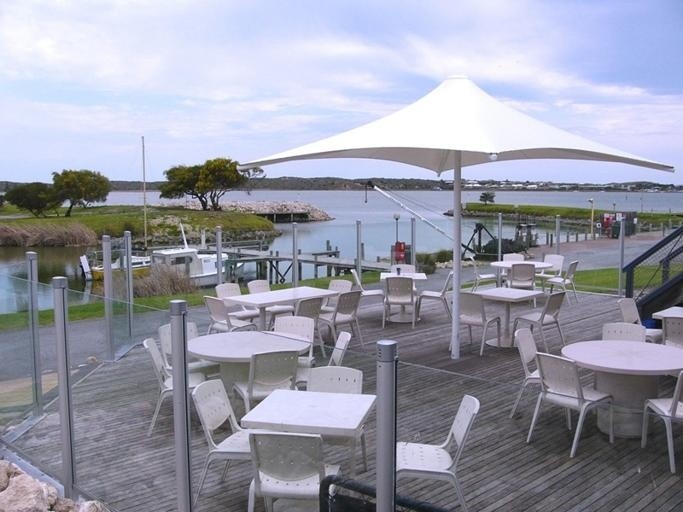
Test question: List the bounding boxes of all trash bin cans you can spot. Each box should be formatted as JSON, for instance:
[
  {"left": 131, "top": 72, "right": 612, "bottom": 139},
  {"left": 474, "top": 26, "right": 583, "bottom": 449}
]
[
  {"left": 515, "top": 222, "right": 539, "bottom": 249},
  {"left": 404, "top": 244, "right": 411, "bottom": 264},
  {"left": 611, "top": 224, "right": 620, "bottom": 238}
]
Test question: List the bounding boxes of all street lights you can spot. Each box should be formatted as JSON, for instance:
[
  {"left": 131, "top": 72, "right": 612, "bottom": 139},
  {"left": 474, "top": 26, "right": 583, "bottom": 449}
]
[
  {"left": 588, "top": 198, "right": 594, "bottom": 237},
  {"left": 395, "top": 212, "right": 401, "bottom": 243}
]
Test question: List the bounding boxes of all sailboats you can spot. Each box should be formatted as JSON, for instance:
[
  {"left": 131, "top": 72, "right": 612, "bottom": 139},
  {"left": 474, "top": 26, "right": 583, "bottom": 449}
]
[{"left": 77, "top": 135, "right": 178, "bottom": 281}]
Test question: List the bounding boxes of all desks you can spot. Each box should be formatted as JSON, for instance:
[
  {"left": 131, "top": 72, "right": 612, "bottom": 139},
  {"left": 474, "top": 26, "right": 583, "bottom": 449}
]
[
  {"left": 234, "top": 387, "right": 378, "bottom": 481},
  {"left": 470, "top": 286, "right": 543, "bottom": 348},
  {"left": 651, "top": 305, "right": 682, "bottom": 321},
  {"left": 487, "top": 259, "right": 553, "bottom": 279},
  {"left": 559, "top": 339, "right": 681, "bottom": 440},
  {"left": 221, "top": 284, "right": 340, "bottom": 330},
  {"left": 184, "top": 328, "right": 313, "bottom": 398},
  {"left": 378, "top": 271, "right": 429, "bottom": 324}
]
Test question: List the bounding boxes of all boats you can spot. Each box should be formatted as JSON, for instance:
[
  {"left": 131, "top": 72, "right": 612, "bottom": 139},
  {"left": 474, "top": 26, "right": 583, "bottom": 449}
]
[
  {"left": 148, "top": 245, "right": 271, "bottom": 289},
  {"left": 357, "top": 179, "right": 541, "bottom": 263}
]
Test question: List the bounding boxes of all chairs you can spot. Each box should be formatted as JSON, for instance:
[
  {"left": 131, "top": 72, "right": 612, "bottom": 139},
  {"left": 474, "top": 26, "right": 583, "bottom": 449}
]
[
  {"left": 291, "top": 296, "right": 328, "bottom": 360},
  {"left": 144, "top": 336, "right": 209, "bottom": 439},
  {"left": 542, "top": 259, "right": 581, "bottom": 307},
  {"left": 505, "top": 262, "right": 538, "bottom": 309},
  {"left": 500, "top": 253, "right": 526, "bottom": 261},
  {"left": 349, "top": 267, "right": 392, "bottom": 322},
  {"left": 615, "top": 296, "right": 663, "bottom": 344},
  {"left": 318, "top": 278, "right": 357, "bottom": 338},
  {"left": 326, "top": 330, "right": 353, "bottom": 367},
  {"left": 525, "top": 350, "right": 618, "bottom": 459},
  {"left": 389, "top": 263, "right": 417, "bottom": 274},
  {"left": 513, "top": 289, "right": 568, "bottom": 354},
  {"left": 639, "top": 370, "right": 681, "bottom": 476},
  {"left": 389, "top": 394, "right": 483, "bottom": 512},
  {"left": 508, "top": 327, "right": 575, "bottom": 432},
  {"left": 316, "top": 472, "right": 454, "bottom": 511},
  {"left": 156, "top": 320, "right": 221, "bottom": 379},
  {"left": 599, "top": 321, "right": 646, "bottom": 343},
  {"left": 201, "top": 293, "right": 258, "bottom": 334},
  {"left": 380, "top": 275, "right": 419, "bottom": 331},
  {"left": 232, "top": 349, "right": 302, "bottom": 413},
  {"left": 535, "top": 253, "right": 566, "bottom": 293},
  {"left": 446, "top": 290, "right": 503, "bottom": 357},
  {"left": 272, "top": 314, "right": 316, "bottom": 370},
  {"left": 467, "top": 256, "right": 499, "bottom": 292},
  {"left": 245, "top": 279, "right": 295, "bottom": 326},
  {"left": 188, "top": 377, "right": 254, "bottom": 510},
  {"left": 660, "top": 316, "right": 681, "bottom": 348},
  {"left": 298, "top": 363, "right": 371, "bottom": 480},
  {"left": 245, "top": 430, "right": 342, "bottom": 509},
  {"left": 317, "top": 289, "right": 367, "bottom": 353},
  {"left": 418, "top": 269, "right": 456, "bottom": 323},
  {"left": 213, "top": 282, "right": 264, "bottom": 327}
]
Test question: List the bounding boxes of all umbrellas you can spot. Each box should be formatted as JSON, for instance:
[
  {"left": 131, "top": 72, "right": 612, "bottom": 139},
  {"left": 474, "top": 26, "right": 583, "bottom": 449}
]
[{"left": 236, "top": 74, "right": 675, "bottom": 360}]
